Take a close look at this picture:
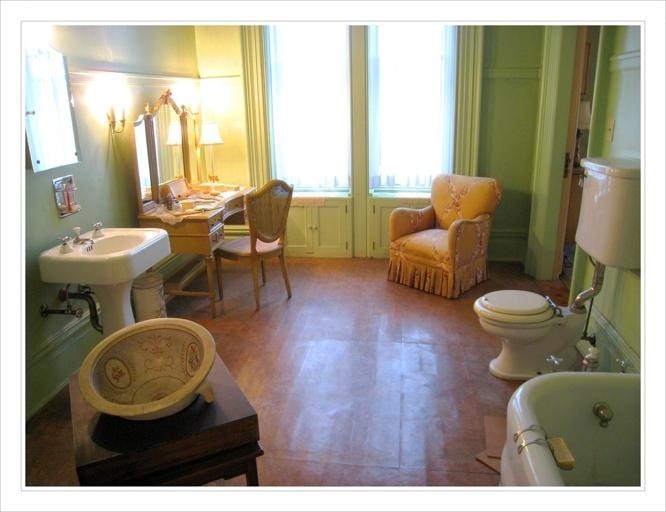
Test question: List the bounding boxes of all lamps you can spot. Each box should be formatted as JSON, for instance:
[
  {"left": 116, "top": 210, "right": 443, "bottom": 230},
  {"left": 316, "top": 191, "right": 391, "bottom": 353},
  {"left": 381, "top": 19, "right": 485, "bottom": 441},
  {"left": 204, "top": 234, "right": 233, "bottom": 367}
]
[
  {"left": 574, "top": 100, "right": 590, "bottom": 168},
  {"left": 198, "top": 122, "right": 224, "bottom": 184},
  {"left": 166, "top": 124, "right": 182, "bottom": 179}
]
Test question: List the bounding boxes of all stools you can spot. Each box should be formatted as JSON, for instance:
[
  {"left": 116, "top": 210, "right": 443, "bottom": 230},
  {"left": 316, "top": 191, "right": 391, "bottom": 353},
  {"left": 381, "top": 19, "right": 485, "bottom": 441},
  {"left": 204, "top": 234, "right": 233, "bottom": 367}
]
[{"left": 67, "top": 352, "right": 264, "bottom": 486}]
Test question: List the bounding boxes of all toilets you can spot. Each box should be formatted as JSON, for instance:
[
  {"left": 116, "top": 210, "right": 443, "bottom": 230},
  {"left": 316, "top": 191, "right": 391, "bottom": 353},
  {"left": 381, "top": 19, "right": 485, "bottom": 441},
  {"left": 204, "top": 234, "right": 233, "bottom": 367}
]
[{"left": 473, "top": 157, "right": 640, "bottom": 381}]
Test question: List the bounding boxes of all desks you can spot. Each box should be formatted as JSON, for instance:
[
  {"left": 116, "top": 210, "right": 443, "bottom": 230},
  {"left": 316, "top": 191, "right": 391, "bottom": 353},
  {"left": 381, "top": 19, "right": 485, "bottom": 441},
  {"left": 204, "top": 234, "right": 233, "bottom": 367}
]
[
  {"left": 564, "top": 170, "right": 585, "bottom": 246},
  {"left": 138, "top": 185, "right": 256, "bottom": 319}
]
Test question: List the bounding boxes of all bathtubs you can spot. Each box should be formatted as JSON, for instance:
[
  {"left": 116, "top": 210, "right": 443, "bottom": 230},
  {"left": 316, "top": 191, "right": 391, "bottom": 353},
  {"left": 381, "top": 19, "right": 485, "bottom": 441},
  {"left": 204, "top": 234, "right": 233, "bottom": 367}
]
[{"left": 499, "top": 373, "right": 638, "bottom": 486}]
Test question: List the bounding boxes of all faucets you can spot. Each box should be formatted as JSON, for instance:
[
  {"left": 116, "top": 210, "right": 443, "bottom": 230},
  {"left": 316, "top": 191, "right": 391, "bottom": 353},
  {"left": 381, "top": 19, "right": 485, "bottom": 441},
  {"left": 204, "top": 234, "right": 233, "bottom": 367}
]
[{"left": 72, "top": 227, "right": 95, "bottom": 244}]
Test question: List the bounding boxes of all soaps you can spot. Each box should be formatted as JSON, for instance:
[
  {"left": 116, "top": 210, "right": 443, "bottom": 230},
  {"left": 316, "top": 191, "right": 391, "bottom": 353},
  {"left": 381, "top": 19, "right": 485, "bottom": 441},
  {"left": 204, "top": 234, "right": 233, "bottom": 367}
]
[{"left": 548, "top": 438, "right": 576, "bottom": 468}]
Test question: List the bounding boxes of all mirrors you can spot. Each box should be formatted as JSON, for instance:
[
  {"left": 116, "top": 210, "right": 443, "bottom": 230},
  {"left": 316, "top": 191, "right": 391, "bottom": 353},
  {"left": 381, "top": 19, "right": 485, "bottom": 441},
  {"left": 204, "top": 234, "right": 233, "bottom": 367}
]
[
  {"left": 23, "top": 45, "right": 79, "bottom": 174},
  {"left": 130, "top": 88, "right": 200, "bottom": 215}
]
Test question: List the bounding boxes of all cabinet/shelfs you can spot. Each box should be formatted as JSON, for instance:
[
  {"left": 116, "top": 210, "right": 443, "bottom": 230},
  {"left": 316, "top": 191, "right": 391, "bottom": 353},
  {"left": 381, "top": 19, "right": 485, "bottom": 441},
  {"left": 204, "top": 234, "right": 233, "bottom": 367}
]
[
  {"left": 365, "top": 197, "right": 431, "bottom": 259},
  {"left": 284, "top": 198, "right": 353, "bottom": 258}
]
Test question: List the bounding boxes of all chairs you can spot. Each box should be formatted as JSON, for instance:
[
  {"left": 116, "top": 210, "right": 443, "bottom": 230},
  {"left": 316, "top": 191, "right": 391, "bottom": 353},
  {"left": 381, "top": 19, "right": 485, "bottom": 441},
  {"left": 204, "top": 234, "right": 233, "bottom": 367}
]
[
  {"left": 386, "top": 173, "right": 502, "bottom": 299},
  {"left": 214, "top": 179, "right": 294, "bottom": 311}
]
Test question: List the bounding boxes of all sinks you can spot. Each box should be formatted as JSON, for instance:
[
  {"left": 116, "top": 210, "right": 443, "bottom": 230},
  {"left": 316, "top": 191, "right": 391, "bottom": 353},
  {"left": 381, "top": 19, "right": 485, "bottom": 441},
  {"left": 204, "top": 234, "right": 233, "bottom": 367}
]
[{"left": 39, "top": 226, "right": 171, "bottom": 287}]
